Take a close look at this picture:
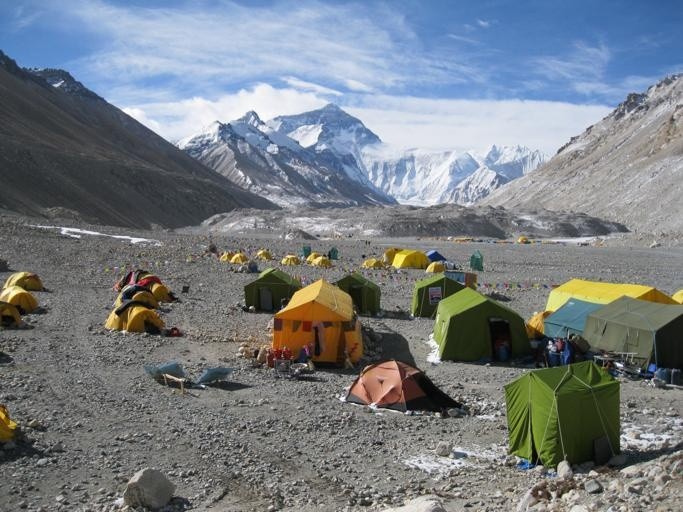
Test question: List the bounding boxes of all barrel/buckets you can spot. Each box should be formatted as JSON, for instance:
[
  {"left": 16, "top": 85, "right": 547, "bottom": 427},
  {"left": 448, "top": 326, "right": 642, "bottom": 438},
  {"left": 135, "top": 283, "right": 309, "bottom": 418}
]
[
  {"left": 670, "top": 368, "right": 681, "bottom": 385},
  {"left": 655, "top": 367, "right": 670, "bottom": 384}
]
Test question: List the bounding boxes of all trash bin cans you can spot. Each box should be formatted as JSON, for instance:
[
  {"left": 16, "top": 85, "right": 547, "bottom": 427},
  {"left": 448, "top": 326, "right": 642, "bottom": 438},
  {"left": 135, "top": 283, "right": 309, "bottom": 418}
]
[{"left": 654, "top": 368, "right": 681, "bottom": 384}]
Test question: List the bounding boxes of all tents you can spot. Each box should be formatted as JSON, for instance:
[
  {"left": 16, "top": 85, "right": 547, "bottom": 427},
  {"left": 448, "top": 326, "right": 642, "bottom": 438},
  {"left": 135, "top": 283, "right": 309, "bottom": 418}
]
[
  {"left": 519, "top": 235, "right": 530, "bottom": 243},
  {"left": 332, "top": 270, "right": 381, "bottom": 316},
  {"left": 0, "top": 286, "right": 38, "bottom": 314},
  {"left": 217, "top": 236, "right": 338, "bottom": 273},
  {"left": 3, "top": 272, "right": 43, "bottom": 291},
  {"left": 425, "top": 261, "right": 446, "bottom": 272},
  {"left": 345, "top": 359, "right": 461, "bottom": 410},
  {"left": 362, "top": 247, "right": 446, "bottom": 268},
  {"left": 502, "top": 359, "right": 620, "bottom": 469},
  {"left": 0, "top": 299, "right": 21, "bottom": 327},
  {"left": 412, "top": 270, "right": 683, "bottom": 379},
  {"left": 272, "top": 278, "right": 363, "bottom": 370},
  {"left": 244, "top": 267, "right": 302, "bottom": 312},
  {"left": 0, "top": 404, "right": 20, "bottom": 444},
  {"left": 105, "top": 269, "right": 172, "bottom": 333}
]
